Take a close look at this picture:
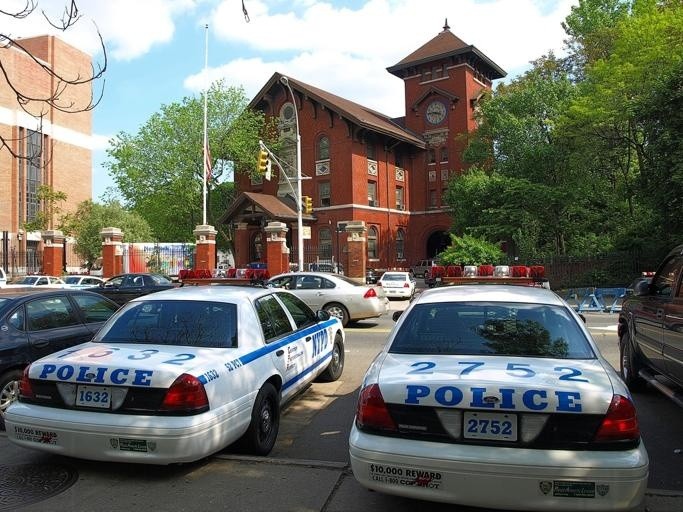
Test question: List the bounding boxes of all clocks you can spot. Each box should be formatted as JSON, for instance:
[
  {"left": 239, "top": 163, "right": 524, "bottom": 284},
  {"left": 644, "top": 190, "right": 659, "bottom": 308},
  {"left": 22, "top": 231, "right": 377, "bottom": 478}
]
[{"left": 422, "top": 97, "right": 448, "bottom": 127}]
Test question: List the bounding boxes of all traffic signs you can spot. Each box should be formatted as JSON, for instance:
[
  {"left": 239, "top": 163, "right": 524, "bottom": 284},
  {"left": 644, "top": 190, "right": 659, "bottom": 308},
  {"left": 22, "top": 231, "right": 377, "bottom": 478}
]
[{"left": 265, "top": 159, "right": 272, "bottom": 181}]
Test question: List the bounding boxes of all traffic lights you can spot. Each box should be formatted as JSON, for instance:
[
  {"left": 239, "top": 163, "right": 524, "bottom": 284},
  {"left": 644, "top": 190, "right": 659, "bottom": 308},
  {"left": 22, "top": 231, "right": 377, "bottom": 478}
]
[
  {"left": 306, "top": 197, "right": 314, "bottom": 213},
  {"left": 258, "top": 151, "right": 267, "bottom": 172}
]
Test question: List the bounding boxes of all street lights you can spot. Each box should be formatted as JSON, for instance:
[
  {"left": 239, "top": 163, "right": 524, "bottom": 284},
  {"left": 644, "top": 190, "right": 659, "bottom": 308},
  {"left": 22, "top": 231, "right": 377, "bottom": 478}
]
[{"left": 278, "top": 75, "right": 304, "bottom": 271}]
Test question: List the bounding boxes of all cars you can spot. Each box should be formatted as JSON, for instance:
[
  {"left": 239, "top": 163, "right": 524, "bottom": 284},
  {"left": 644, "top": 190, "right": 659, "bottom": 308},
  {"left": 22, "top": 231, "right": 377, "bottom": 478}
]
[
  {"left": 7, "top": 271, "right": 64, "bottom": 286},
  {"left": 344, "top": 265, "right": 649, "bottom": 511},
  {"left": 225, "top": 261, "right": 270, "bottom": 281},
  {"left": 0, "top": 267, "right": 7, "bottom": 283},
  {"left": 79, "top": 273, "right": 194, "bottom": 313},
  {"left": 265, "top": 270, "right": 389, "bottom": 327},
  {"left": 3, "top": 268, "right": 344, "bottom": 469},
  {"left": 375, "top": 271, "right": 417, "bottom": 301},
  {"left": 309, "top": 260, "right": 344, "bottom": 276},
  {"left": 624, "top": 270, "right": 658, "bottom": 300},
  {"left": 60, "top": 271, "right": 108, "bottom": 288},
  {"left": 1, "top": 285, "right": 124, "bottom": 438},
  {"left": 365, "top": 271, "right": 386, "bottom": 284}
]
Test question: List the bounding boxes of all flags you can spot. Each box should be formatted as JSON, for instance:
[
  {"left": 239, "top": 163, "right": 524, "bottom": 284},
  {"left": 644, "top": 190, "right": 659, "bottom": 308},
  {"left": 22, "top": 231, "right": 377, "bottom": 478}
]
[{"left": 206, "top": 137, "right": 211, "bottom": 185}]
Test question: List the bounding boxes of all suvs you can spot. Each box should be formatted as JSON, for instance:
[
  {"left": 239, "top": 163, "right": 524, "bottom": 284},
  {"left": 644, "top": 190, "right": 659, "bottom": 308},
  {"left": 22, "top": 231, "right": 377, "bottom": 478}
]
[
  {"left": 365, "top": 266, "right": 375, "bottom": 277},
  {"left": 616, "top": 245, "right": 682, "bottom": 412},
  {"left": 412, "top": 259, "right": 440, "bottom": 279}
]
